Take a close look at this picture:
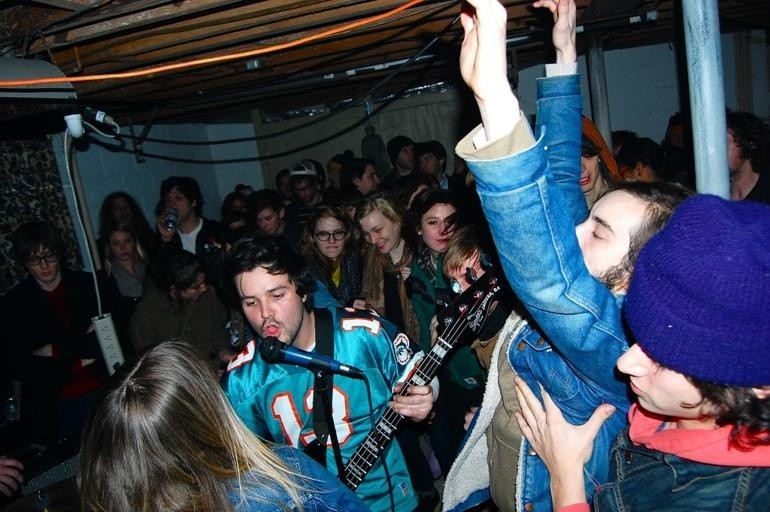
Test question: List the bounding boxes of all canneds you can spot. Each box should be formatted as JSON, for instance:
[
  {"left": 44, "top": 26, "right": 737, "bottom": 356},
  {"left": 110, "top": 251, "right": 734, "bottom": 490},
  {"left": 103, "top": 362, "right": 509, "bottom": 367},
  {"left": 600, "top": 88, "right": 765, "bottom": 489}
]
[{"left": 165, "top": 208, "right": 177, "bottom": 232}]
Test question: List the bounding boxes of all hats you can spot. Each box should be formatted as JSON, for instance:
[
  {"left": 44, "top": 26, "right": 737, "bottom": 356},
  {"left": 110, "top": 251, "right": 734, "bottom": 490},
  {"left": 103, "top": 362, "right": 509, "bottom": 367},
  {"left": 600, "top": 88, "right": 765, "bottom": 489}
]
[
  {"left": 623, "top": 193, "right": 770, "bottom": 386},
  {"left": 289, "top": 159, "right": 318, "bottom": 175}
]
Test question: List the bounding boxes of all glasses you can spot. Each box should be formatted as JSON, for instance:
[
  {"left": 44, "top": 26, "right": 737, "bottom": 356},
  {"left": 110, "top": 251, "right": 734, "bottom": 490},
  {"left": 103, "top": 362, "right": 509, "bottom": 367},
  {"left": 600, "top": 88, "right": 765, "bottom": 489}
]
[
  {"left": 315, "top": 231, "right": 347, "bottom": 241},
  {"left": 25, "top": 252, "right": 57, "bottom": 266}
]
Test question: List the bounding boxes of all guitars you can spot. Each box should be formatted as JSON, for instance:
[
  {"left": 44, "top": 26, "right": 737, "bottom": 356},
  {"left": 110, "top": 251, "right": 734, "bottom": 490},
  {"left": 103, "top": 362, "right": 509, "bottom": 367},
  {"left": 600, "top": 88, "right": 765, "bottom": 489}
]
[{"left": 338, "top": 261, "right": 506, "bottom": 493}]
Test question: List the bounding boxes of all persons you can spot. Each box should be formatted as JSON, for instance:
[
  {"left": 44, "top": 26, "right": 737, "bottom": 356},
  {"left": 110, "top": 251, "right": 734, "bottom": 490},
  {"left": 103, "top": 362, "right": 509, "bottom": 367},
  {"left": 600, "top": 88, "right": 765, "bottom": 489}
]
[{"left": 1, "top": 1, "right": 770, "bottom": 512}]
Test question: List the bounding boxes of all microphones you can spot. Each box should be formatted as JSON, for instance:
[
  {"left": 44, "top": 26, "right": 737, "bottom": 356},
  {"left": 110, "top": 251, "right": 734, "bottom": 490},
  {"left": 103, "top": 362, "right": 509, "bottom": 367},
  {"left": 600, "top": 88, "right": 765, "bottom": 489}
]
[{"left": 258, "top": 336, "right": 364, "bottom": 380}]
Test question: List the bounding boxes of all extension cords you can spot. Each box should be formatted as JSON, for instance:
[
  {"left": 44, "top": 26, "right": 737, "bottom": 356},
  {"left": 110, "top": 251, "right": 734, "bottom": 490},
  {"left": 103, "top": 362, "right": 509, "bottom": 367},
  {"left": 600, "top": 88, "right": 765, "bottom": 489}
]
[{"left": 91, "top": 312, "right": 125, "bottom": 377}]
